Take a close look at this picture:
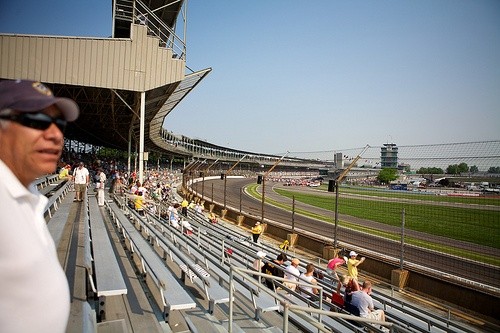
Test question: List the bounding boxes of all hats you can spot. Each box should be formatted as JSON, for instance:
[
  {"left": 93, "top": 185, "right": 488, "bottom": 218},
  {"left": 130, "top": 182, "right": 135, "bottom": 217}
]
[
  {"left": 349, "top": 251, "right": 358, "bottom": 255},
  {"left": 79, "top": 162, "right": 84, "bottom": 165},
  {"left": 255, "top": 221, "right": 260, "bottom": 225},
  {"left": 292, "top": 259, "right": 300, "bottom": 264},
  {"left": 343, "top": 256, "right": 348, "bottom": 264},
  {"left": 0, "top": 79, "right": 80, "bottom": 121},
  {"left": 257, "top": 251, "right": 267, "bottom": 257}
]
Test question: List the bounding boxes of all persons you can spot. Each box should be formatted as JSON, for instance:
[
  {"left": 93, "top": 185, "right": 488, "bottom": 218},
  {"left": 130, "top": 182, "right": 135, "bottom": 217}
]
[
  {"left": 169, "top": 204, "right": 180, "bottom": 227},
  {"left": 351, "top": 280, "right": 385, "bottom": 323},
  {"left": 326, "top": 256, "right": 348, "bottom": 282},
  {"left": 261, "top": 252, "right": 288, "bottom": 291},
  {"left": 95, "top": 167, "right": 106, "bottom": 207},
  {"left": 252, "top": 251, "right": 267, "bottom": 280},
  {"left": 252, "top": 222, "right": 261, "bottom": 244},
  {"left": 348, "top": 251, "right": 365, "bottom": 281},
  {"left": 59, "top": 164, "right": 71, "bottom": 184},
  {"left": 72, "top": 160, "right": 88, "bottom": 204},
  {"left": 0, "top": 80, "right": 79, "bottom": 333},
  {"left": 283, "top": 259, "right": 301, "bottom": 291},
  {"left": 345, "top": 278, "right": 361, "bottom": 304},
  {"left": 57, "top": 149, "right": 378, "bottom": 217},
  {"left": 298, "top": 264, "right": 326, "bottom": 301}
]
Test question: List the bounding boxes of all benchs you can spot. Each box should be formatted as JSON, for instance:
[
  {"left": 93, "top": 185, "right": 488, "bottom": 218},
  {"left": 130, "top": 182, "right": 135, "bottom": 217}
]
[{"left": 30, "top": 174, "right": 485, "bottom": 333}]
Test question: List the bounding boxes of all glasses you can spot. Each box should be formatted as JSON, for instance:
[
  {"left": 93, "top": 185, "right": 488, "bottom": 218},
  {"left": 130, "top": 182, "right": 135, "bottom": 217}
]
[{"left": 0, "top": 112, "right": 67, "bottom": 133}]
